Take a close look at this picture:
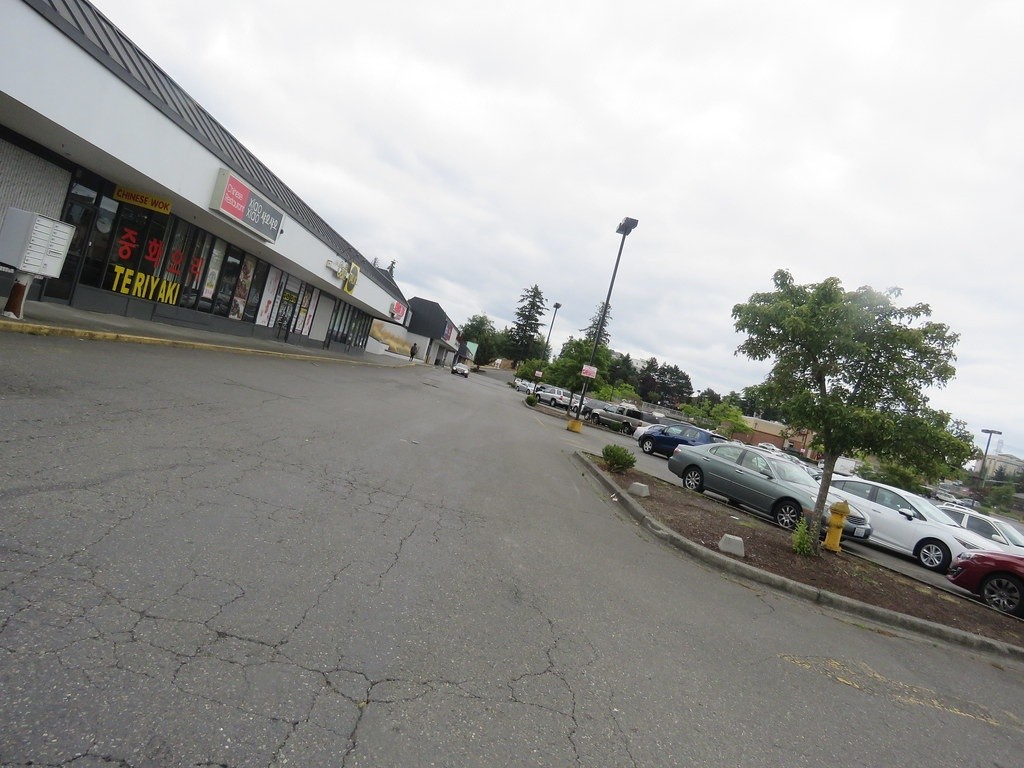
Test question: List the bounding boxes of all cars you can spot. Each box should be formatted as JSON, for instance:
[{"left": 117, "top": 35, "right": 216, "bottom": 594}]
[
  {"left": 516, "top": 381, "right": 535, "bottom": 395},
  {"left": 637, "top": 424, "right": 729, "bottom": 459},
  {"left": 756, "top": 442, "right": 783, "bottom": 453},
  {"left": 813, "top": 478, "right": 1007, "bottom": 573},
  {"left": 535, "top": 387, "right": 575, "bottom": 411},
  {"left": 945, "top": 549, "right": 1024, "bottom": 618},
  {"left": 668, "top": 441, "right": 873, "bottom": 543},
  {"left": 513, "top": 377, "right": 1024, "bottom": 553},
  {"left": 451, "top": 362, "right": 470, "bottom": 378}
]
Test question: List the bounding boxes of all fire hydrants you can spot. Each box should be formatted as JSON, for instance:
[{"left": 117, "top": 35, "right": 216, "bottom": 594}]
[{"left": 821, "top": 499, "right": 849, "bottom": 555}]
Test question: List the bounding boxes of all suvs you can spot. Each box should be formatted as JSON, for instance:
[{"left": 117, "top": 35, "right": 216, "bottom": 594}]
[{"left": 591, "top": 403, "right": 660, "bottom": 436}]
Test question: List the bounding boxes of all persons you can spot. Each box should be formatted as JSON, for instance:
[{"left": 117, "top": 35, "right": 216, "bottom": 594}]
[{"left": 408, "top": 343, "right": 417, "bottom": 362}]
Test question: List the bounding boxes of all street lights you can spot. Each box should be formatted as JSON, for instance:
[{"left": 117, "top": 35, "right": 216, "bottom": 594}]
[
  {"left": 575, "top": 215, "right": 639, "bottom": 420},
  {"left": 531, "top": 302, "right": 563, "bottom": 396},
  {"left": 971, "top": 428, "right": 1003, "bottom": 507}
]
